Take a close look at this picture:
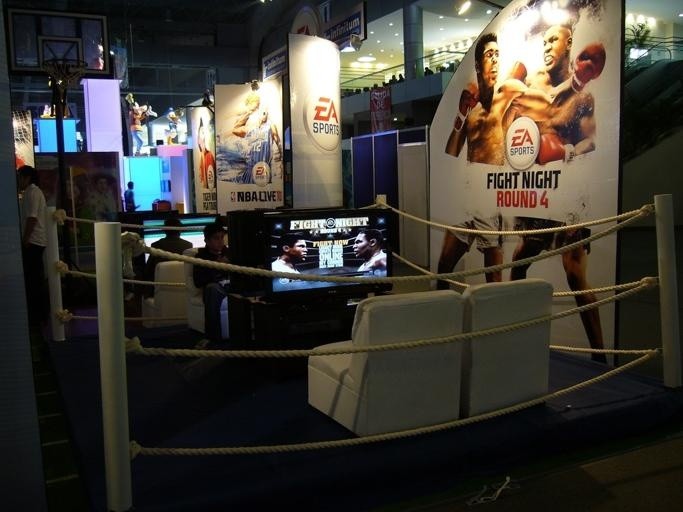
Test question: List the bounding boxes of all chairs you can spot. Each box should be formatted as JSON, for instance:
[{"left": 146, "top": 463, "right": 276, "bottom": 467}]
[
  {"left": 464, "top": 280, "right": 554, "bottom": 421},
  {"left": 307, "top": 290, "right": 465, "bottom": 437},
  {"left": 142, "top": 248, "right": 230, "bottom": 338}
]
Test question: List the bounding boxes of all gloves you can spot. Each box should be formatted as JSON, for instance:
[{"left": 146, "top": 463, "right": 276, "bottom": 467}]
[
  {"left": 537, "top": 133, "right": 575, "bottom": 165},
  {"left": 505, "top": 61, "right": 527, "bottom": 83},
  {"left": 571, "top": 40, "right": 606, "bottom": 92},
  {"left": 453, "top": 83, "right": 480, "bottom": 132}
]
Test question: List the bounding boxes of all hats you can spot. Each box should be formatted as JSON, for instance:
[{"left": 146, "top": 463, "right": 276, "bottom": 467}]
[{"left": 203, "top": 223, "right": 227, "bottom": 241}]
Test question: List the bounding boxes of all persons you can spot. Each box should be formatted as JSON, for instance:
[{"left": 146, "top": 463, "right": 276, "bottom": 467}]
[
  {"left": 15, "top": 164, "right": 49, "bottom": 328},
  {"left": 270, "top": 231, "right": 310, "bottom": 284},
  {"left": 189, "top": 223, "right": 232, "bottom": 348},
  {"left": 229, "top": 101, "right": 284, "bottom": 184},
  {"left": 133, "top": 214, "right": 194, "bottom": 300},
  {"left": 127, "top": 101, "right": 149, "bottom": 156},
  {"left": 503, "top": 23, "right": 609, "bottom": 368},
  {"left": 123, "top": 180, "right": 136, "bottom": 212},
  {"left": 166, "top": 107, "right": 182, "bottom": 147},
  {"left": 196, "top": 118, "right": 216, "bottom": 193},
  {"left": 350, "top": 228, "right": 386, "bottom": 277},
  {"left": 64, "top": 174, "right": 118, "bottom": 242},
  {"left": 433, "top": 33, "right": 607, "bottom": 291}
]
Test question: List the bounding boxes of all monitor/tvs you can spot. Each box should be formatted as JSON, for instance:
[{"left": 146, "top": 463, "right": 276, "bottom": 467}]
[
  {"left": 140, "top": 213, "right": 222, "bottom": 266},
  {"left": 226, "top": 210, "right": 264, "bottom": 295},
  {"left": 261, "top": 208, "right": 393, "bottom": 312}
]
